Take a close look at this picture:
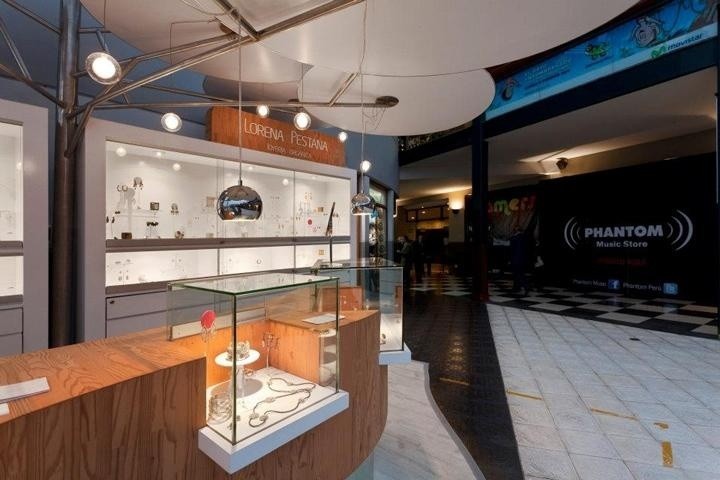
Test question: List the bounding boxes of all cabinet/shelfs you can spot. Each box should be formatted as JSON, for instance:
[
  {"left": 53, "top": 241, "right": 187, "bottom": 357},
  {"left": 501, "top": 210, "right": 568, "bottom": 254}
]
[
  {"left": 0, "top": 97, "right": 53, "bottom": 357},
  {"left": 2, "top": 257, "right": 412, "bottom": 480},
  {"left": 72, "top": 114, "right": 363, "bottom": 340}
]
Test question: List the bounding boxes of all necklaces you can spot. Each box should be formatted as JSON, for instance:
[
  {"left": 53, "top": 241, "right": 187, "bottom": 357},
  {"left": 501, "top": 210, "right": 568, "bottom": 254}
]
[
  {"left": 268, "top": 377, "right": 316, "bottom": 392},
  {"left": 247, "top": 389, "right": 311, "bottom": 426}
]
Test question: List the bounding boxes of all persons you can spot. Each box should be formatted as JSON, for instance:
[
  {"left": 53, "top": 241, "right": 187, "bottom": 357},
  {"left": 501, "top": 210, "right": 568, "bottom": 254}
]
[{"left": 393, "top": 223, "right": 536, "bottom": 299}]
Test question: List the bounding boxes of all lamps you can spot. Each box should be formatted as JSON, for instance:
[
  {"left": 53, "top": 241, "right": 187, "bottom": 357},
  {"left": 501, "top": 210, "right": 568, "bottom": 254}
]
[
  {"left": 351, "top": 103, "right": 376, "bottom": 217},
  {"left": 293, "top": 109, "right": 312, "bottom": 133},
  {"left": 161, "top": 110, "right": 183, "bottom": 136},
  {"left": 256, "top": 103, "right": 272, "bottom": 120},
  {"left": 83, "top": 45, "right": 125, "bottom": 90},
  {"left": 358, "top": 157, "right": 374, "bottom": 175},
  {"left": 217, "top": 0, "right": 266, "bottom": 224},
  {"left": 348, "top": 70, "right": 371, "bottom": 208},
  {"left": 554, "top": 157, "right": 569, "bottom": 170},
  {"left": 337, "top": 130, "right": 348, "bottom": 144}
]
